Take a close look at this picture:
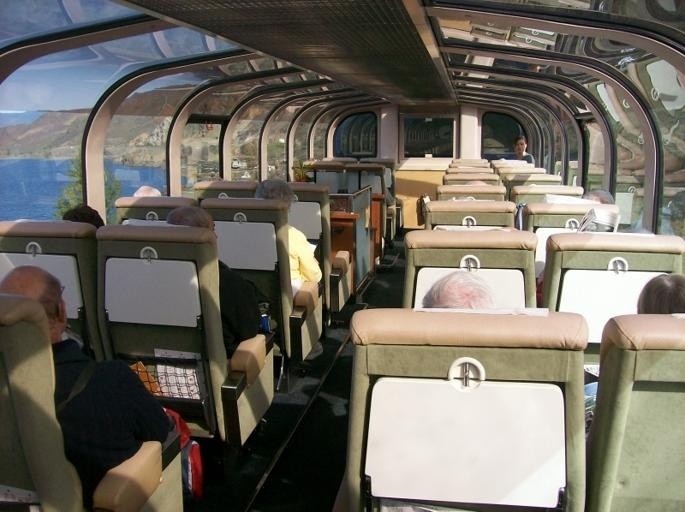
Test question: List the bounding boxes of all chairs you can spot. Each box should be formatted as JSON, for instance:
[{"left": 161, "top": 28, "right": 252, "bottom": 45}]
[{"left": 0, "top": 153, "right": 685, "bottom": 512}]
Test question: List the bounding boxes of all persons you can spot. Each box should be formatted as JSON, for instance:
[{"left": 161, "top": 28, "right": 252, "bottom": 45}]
[
  {"left": 580, "top": 188, "right": 615, "bottom": 206},
  {"left": 419, "top": 270, "right": 499, "bottom": 313},
  {"left": 253, "top": 178, "right": 324, "bottom": 360},
  {"left": 513, "top": 137, "right": 534, "bottom": 164},
  {"left": 165, "top": 206, "right": 260, "bottom": 358},
  {"left": 584, "top": 272, "right": 685, "bottom": 426},
  {"left": 0, "top": 265, "right": 203, "bottom": 512},
  {"left": 61, "top": 203, "right": 105, "bottom": 229},
  {"left": 130, "top": 185, "right": 162, "bottom": 198}
]
